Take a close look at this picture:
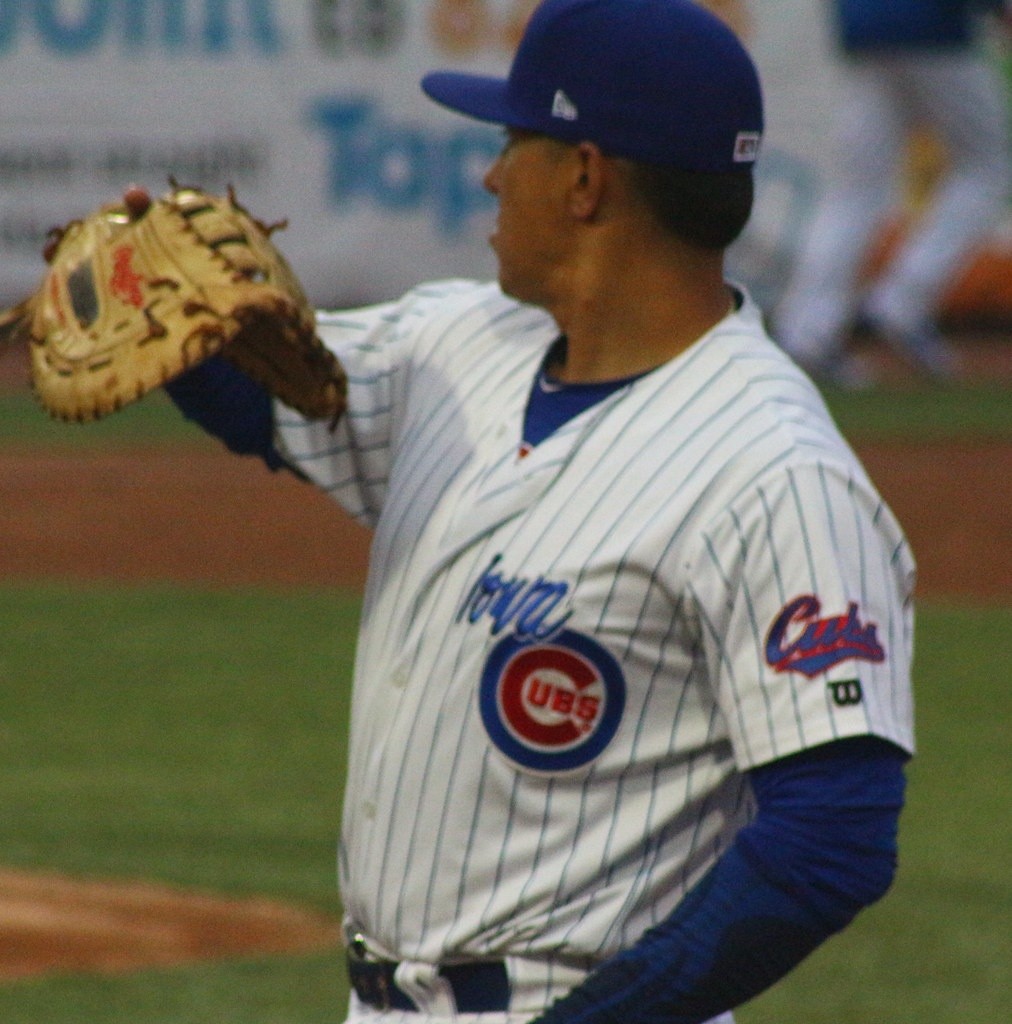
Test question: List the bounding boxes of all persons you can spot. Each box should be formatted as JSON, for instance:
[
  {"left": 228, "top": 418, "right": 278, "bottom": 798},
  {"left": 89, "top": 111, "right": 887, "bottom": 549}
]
[
  {"left": 762, "top": 0, "right": 1012, "bottom": 382},
  {"left": 41, "top": 1, "right": 915, "bottom": 1024}
]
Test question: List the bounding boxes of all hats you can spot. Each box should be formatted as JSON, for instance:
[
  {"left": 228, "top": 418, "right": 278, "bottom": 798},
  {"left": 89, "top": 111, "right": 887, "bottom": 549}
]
[{"left": 422, "top": 0, "right": 764, "bottom": 175}]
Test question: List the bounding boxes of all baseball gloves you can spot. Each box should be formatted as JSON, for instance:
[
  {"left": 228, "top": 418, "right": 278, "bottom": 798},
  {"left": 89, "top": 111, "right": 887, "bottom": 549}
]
[{"left": 24, "top": 181, "right": 351, "bottom": 424}]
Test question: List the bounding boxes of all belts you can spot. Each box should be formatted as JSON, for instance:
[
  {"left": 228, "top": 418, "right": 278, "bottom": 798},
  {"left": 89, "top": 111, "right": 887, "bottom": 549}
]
[{"left": 345, "top": 944, "right": 509, "bottom": 1012}]
